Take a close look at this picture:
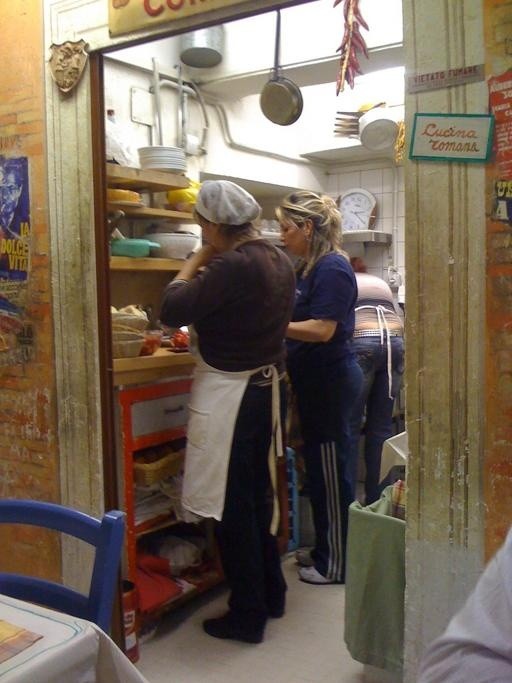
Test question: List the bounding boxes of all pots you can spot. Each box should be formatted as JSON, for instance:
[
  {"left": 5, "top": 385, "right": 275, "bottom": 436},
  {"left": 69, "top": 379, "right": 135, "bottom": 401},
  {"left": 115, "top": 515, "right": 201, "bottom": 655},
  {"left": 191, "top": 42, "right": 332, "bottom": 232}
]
[{"left": 258, "top": 9, "right": 305, "bottom": 126}]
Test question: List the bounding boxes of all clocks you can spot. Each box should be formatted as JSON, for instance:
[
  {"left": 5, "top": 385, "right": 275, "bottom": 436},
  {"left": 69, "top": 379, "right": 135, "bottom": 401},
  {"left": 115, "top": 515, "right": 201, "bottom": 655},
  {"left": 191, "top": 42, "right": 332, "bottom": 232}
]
[{"left": 337, "top": 185, "right": 376, "bottom": 230}]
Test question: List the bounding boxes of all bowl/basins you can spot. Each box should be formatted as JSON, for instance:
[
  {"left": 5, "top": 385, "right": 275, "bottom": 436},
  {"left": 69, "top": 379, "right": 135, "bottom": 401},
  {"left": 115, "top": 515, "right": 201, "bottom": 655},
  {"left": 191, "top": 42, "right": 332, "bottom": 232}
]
[{"left": 145, "top": 233, "right": 200, "bottom": 260}]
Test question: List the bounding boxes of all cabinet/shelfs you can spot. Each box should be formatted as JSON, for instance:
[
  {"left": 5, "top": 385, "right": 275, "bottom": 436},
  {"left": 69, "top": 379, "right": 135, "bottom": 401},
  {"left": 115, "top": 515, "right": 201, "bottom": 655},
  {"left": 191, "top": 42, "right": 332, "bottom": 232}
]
[
  {"left": 121, "top": 381, "right": 228, "bottom": 628},
  {"left": 105, "top": 161, "right": 199, "bottom": 388}
]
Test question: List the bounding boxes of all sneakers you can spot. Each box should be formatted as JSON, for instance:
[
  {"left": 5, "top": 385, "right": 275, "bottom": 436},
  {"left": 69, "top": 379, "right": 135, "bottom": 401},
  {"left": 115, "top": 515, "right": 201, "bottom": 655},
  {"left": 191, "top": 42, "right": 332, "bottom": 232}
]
[
  {"left": 201, "top": 591, "right": 289, "bottom": 644},
  {"left": 295, "top": 549, "right": 316, "bottom": 566},
  {"left": 298, "top": 565, "right": 341, "bottom": 586}
]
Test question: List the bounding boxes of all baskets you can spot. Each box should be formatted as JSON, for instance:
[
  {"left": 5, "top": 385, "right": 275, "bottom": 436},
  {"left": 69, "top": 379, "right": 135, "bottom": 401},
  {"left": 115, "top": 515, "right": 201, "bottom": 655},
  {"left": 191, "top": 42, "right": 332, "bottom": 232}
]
[
  {"left": 111, "top": 312, "right": 151, "bottom": 358},
  {"left": 132, "top": 445, "right": 190, "bottom": 490}
]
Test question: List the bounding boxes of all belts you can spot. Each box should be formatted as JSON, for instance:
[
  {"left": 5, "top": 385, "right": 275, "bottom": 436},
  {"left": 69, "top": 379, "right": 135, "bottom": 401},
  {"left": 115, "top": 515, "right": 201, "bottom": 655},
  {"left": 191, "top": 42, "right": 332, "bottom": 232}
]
[{"left": 353, "top": 327, "right": 403, "bottom": 337}]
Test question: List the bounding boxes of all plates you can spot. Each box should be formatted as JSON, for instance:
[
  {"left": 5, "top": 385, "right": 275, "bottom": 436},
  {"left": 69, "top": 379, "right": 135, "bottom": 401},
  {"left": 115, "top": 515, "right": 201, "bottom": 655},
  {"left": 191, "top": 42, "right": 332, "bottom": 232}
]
[{"left": 137, "top": 146, "right": 187, "bottom": 175}]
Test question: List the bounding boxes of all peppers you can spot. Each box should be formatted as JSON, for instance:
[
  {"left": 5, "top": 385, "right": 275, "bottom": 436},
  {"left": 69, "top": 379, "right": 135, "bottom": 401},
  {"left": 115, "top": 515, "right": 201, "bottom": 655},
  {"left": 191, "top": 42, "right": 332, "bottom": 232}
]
[{"left": 333, "top": 0, "right": 370, "bottom": 98}]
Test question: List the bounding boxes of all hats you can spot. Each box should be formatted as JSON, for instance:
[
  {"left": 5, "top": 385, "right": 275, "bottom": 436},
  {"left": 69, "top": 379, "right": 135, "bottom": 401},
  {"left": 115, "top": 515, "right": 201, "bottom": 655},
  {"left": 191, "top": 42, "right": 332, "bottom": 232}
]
[{"left": 195, "top": 179, "right": 262, "bottom": 227}]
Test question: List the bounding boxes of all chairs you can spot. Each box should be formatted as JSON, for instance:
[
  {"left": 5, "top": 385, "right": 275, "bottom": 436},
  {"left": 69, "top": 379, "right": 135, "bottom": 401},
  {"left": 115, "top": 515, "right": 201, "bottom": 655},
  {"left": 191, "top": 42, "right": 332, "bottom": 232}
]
[{"left": 1, "top": 496, "right": 127, "bottom": 641}]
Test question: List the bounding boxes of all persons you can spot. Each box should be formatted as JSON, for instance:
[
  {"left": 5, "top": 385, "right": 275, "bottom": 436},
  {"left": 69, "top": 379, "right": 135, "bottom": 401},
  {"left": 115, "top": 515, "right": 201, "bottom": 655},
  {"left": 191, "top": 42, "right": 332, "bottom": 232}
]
[
  {"left": 269, "top": 186, "right": 368, "bottom": 586},
  {"left": 344, "top": 254, "right": 407, "bottom": 507},
  {"left": 156, "top": 177, "right": 298, "bottom": 644}
]
